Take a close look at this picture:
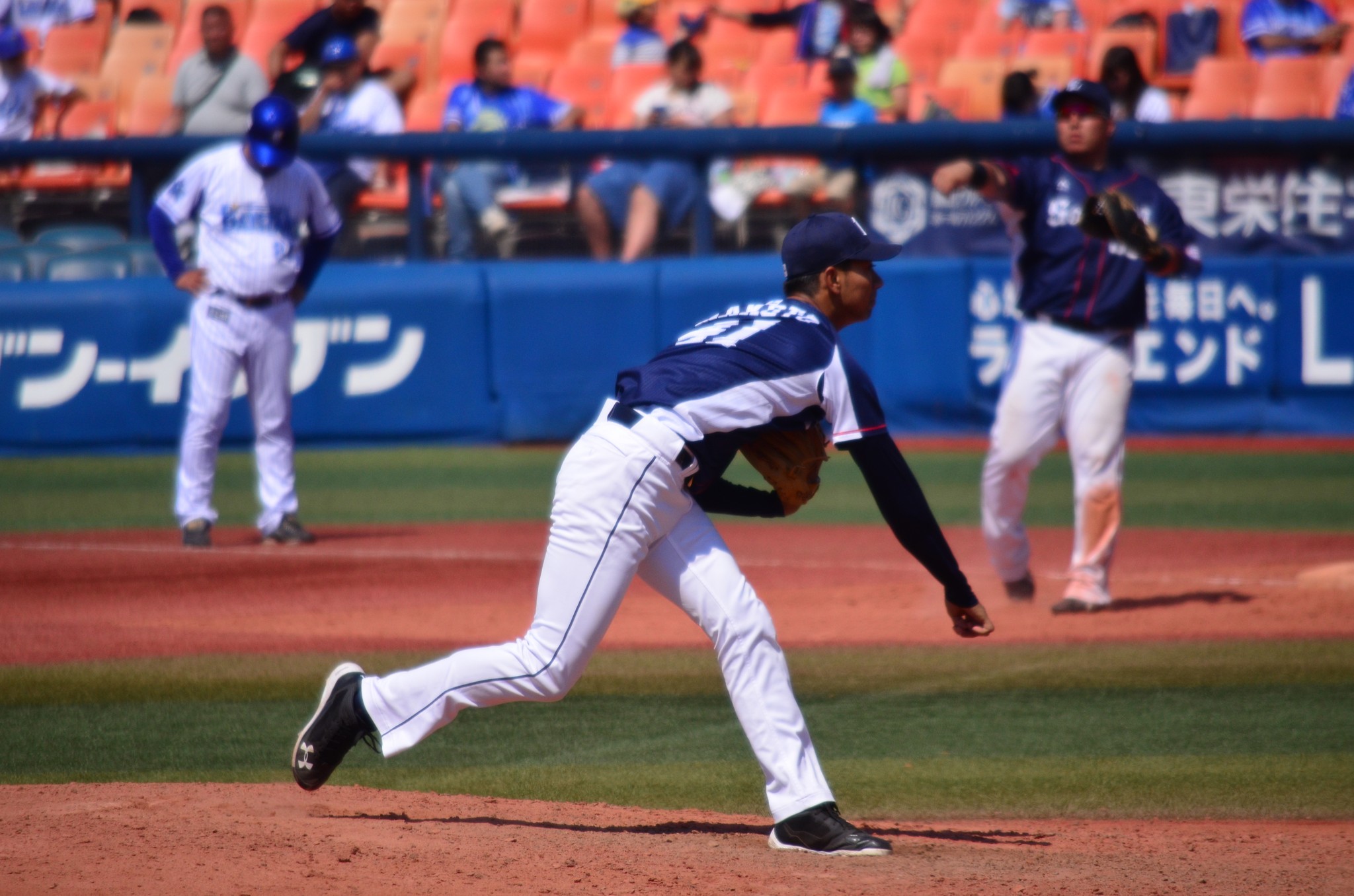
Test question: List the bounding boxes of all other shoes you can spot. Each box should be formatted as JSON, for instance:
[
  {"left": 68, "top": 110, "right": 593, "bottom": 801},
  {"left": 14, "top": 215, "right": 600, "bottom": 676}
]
[
  {"left": 1004, "top": 573, "right": 1033, "bottom": 598},
  {"left": 1051, "top": 597, "right": 1101, "bottom": 613}
]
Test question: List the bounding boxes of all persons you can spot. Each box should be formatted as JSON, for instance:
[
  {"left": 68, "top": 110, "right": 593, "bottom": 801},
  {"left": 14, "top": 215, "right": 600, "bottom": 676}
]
[
  {"left": 141, "top": 92, "right": 343, "bottom": 549},
  {"left": 932, "top": 80, "right": 1186, "bottom": 614},
  {"left": 0, "top": 1, "right": 1353, "bottom": 264},
  {"left": 294, "top": 209, "right": 996, "bottom": 858}
]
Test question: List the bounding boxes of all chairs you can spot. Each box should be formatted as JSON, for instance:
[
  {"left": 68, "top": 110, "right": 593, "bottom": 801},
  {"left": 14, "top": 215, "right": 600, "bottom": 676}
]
[{"left": 0, "top": 1, "right": 1354, "bottom": 280}]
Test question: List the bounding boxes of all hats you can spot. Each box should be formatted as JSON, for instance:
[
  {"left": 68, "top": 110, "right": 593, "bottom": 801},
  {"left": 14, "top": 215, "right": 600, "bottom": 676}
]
[
  {"left": 1050, "top": 80, "right": 1111, "bottom": 118},
  {"left": 781, "top": 213, "right": 903, "bottom": 280},
  {"left": 0, "top": 29, "right": 27, "bottom": 61},
  {"left": 321, "top": 36, "right": 355, "bottom": 66}
]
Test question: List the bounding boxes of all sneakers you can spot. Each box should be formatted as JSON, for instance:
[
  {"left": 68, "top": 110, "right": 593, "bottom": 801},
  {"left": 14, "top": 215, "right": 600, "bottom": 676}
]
[
  {"left": 292, "top": 662, "right": 383, "bottom": 792},
  {"left": 182, "top": 518, "right": 213, "bottom": 549},
  {"left": 766, "top": 803, "right": 893, "bottom": 857},
  {"left": 263, "top": 515, "right": 317, "bottom": 544}
]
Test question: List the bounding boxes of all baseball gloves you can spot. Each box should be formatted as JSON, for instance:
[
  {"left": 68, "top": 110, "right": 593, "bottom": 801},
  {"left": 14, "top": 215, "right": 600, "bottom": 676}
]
[
  {"left": 1081, "top": 187, "right": 1160, "bottom": 258},
  {"left": 735, "top": 409, "right": 829, "bottom": 508}
]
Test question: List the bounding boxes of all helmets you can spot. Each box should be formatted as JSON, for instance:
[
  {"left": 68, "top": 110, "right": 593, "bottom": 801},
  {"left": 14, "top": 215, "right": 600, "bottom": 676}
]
[{"left": 251, "top": 96, "right": 303, "bottom": 167}]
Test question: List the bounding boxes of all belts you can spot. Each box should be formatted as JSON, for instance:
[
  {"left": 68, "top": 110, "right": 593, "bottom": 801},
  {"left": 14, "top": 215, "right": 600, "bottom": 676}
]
[
  {"left": 215, "top": 290, "right": 275, "bottom": 310},
  {"left": 609, "top": 402, "right": 695, "bottom": 491},
  {"left": 1026, "top": 311, "right": 1101, "bottom": 335}
]
